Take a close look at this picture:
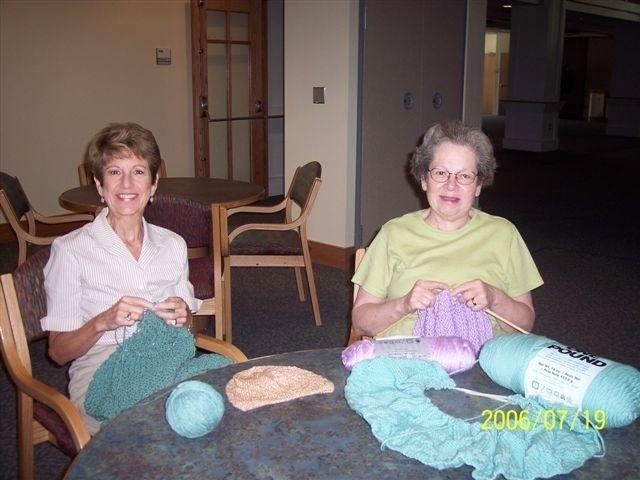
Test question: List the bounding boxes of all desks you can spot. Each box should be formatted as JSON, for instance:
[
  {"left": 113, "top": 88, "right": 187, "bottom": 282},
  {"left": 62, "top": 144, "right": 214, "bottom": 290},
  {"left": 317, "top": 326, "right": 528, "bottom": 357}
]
[{"left": 62, "top": 345, "right": 640, "bottom": 480}]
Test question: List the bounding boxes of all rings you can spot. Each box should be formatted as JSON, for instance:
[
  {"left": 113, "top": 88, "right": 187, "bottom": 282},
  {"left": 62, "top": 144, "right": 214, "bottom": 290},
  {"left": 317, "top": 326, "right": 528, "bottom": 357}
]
[
  {"left": 173, "top": 319, "right": 178, "bottom": 327},
  {"left": 124, "top": 312, "right": 132, "bottom": 320},
  {"left": 470, "top": 297, "right": 479, "bottom": 307}
]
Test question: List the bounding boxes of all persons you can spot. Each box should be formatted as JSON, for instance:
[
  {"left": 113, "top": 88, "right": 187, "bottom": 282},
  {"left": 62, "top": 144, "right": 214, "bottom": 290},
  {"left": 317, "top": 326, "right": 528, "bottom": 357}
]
[
  {"left": 38, "top": 119, "right": 205, "bottom": 440},
  {"left": 347, "top": 117, "right": 547, "bottom": 346}
]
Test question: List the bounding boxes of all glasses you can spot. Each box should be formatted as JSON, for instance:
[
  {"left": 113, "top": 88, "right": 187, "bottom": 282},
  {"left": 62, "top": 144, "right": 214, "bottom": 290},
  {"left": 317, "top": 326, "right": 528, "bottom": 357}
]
[{"left": 426, "top": 167, "right": 481, "bottom": 185}]
